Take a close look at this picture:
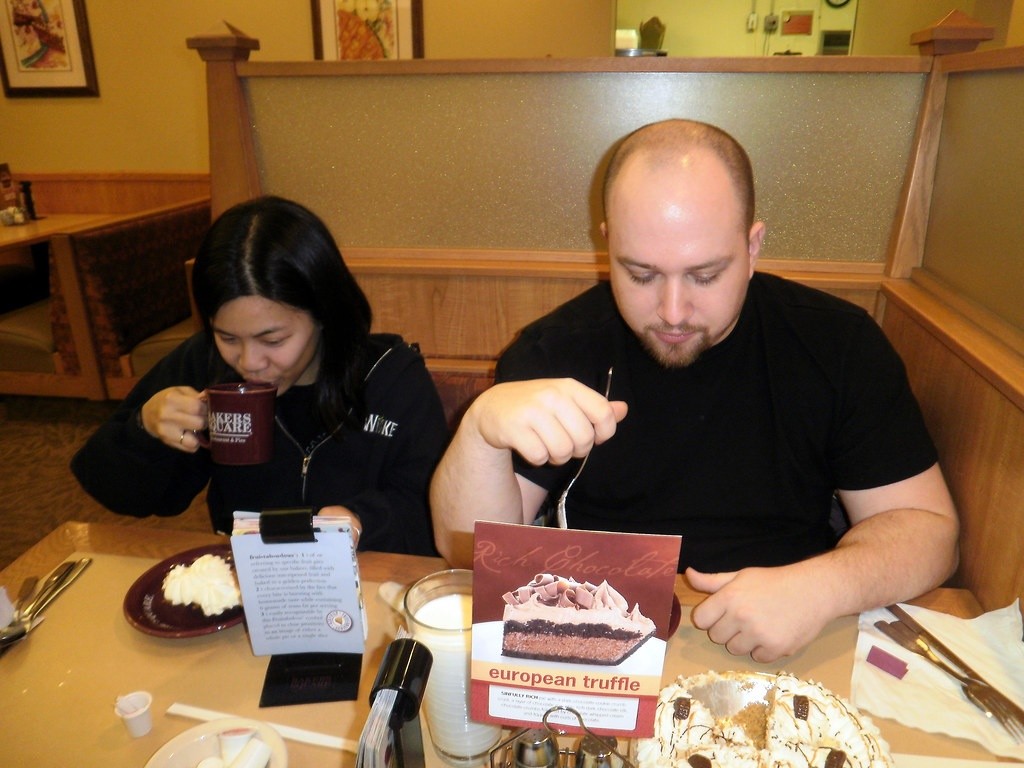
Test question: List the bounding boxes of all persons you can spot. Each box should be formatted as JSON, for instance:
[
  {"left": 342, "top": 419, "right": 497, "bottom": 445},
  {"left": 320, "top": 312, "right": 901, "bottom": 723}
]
[
  {"left": 71, "top": 196, "right": 449, "bottom": 558},
  {"left": 429, "top": 120, "right": 959, "bottom": 664}
]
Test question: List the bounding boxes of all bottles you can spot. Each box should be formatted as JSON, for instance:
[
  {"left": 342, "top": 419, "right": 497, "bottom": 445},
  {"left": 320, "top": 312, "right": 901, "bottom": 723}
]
[{"left": 511, "top": 725, "right": 626, "bottom": 768}]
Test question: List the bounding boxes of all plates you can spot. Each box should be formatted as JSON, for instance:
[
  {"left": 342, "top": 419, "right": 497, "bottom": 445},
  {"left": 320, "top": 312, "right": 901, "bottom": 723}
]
[{"left": 123, "top": 542, "right": 246, "bottom": 639}]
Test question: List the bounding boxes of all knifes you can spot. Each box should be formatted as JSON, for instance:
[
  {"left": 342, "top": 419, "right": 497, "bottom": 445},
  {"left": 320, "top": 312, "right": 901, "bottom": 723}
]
[{"left": 885, "top": 603, "right": 1024, "bottom": 727}]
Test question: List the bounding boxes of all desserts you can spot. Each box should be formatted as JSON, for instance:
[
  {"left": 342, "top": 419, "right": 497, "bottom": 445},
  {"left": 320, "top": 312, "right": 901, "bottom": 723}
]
[{"left": 499, "top": 574, "right": 656, "bottom": 665}]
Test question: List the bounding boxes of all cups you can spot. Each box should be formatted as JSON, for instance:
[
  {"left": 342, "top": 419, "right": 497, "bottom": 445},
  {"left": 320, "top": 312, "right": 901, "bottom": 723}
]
[
  {"left": 189, "top": 382, "right": 277, "bottom": 467},
  {"left": 114, "top": 690, "right": 153, "bottom": 738},
  {"left": 403, "top": 568, "right": 505, "bottom": 768}
]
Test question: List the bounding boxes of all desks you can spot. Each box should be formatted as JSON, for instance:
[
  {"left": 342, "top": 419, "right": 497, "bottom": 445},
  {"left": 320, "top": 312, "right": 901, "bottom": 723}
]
[{"left": 0, "top": 214, "right": 118, "bottom": 292}]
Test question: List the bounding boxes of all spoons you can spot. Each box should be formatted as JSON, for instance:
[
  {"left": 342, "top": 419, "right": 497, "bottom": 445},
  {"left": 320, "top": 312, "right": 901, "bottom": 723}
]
[
  {"left": 556, "top": 365, "right": 613, "bottom": 530},
  {"left": 0, "top": 576, "right": 39, "bottom": 647},
  {"left": 889, "top": 621, "right": 1010, "bottom": 722}
]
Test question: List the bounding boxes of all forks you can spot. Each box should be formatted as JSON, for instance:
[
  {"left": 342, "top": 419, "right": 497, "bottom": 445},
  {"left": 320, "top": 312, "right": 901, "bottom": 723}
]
[
  {"left": 874, "top": 620, "right": 1024, "bottom": 745},
  {"left": 1, "top": 562, "right": 75, "bottom": 646}
]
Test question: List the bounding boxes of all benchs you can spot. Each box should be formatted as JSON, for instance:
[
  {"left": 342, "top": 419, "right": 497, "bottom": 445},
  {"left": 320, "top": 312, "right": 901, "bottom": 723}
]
[{"left": 0, "top": 193, "right": 211, "bottom": 400}]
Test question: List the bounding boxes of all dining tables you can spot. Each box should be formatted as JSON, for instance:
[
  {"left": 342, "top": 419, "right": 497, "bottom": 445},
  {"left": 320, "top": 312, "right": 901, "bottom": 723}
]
[{"left": 0, "top": 519, "right": 1024, "bottom": 768}]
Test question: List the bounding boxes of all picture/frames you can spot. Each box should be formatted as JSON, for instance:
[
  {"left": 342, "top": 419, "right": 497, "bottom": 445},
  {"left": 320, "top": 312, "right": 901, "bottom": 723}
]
[
  {"left": 311, "top": 0, "right": 424, "bottom": 62},
  {"left": 0, "top": 0, "right": 100, "bottom": 97}
]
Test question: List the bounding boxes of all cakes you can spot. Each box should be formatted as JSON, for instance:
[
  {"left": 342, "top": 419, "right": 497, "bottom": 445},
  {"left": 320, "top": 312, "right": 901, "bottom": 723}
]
[
  {"left": 161, "top": 553, "right": 241, "bottom": 616},
  {"left": 625, "top": 673, "right": 888, "bottom": 768}
]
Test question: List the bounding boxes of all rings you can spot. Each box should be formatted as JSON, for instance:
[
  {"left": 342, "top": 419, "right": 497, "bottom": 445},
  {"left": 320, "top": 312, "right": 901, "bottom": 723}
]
[
  {"left": 180, "top": 430, "right": 186, "bottom": 444},
  {"left": 353, "top": 526, "right": 359, "bottom": 535}
]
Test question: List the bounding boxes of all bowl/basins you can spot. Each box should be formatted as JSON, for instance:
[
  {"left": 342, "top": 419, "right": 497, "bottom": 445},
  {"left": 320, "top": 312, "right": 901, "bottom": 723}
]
[{"left": 143, "top": 717, "right": 287, "bottom": 768}]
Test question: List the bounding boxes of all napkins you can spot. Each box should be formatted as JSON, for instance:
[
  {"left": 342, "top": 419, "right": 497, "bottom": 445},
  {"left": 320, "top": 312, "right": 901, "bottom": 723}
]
[
  {"left": 0, "top": 587, "right": 45, "bottom": 648},
  {"left": 851, "top": 601, "right": 1024, "bottom": 759}
]
